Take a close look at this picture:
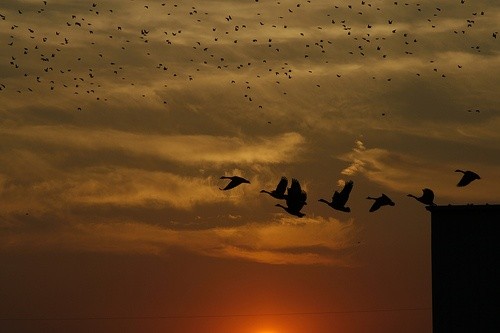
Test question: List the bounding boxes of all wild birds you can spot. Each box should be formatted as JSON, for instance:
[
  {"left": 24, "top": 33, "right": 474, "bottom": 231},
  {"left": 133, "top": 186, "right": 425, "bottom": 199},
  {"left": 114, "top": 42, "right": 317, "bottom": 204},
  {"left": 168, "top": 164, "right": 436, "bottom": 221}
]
[
  {"left": 317, "top": 180, "right": 354, "bottom": 212},
  {"left": 275, "top": 179, "right": 307, "bottom": 219},
  {"left": 455, "top": 170, "right": 481, "bottom": 188},
  {"left": 407, "top": 188, "right": 437, "bottom": 206},
  {"left": 218, "top": 176, "right": 251, "bottom": 191},
  {"left": 260, "top": 176, "right": 288, "bottom": 200},
  {"left": 365, "top": 194, "right": 395, "bottom": 213},
  {"left": 0, "top": 0, "right": 500, "bottom": 124}
]
